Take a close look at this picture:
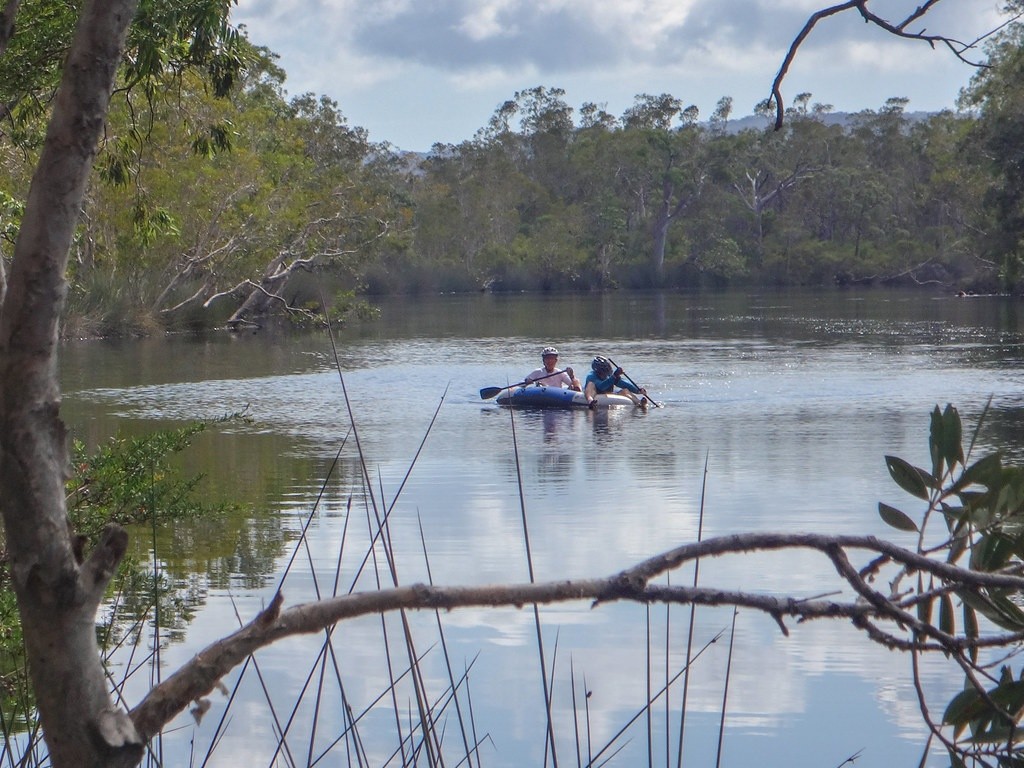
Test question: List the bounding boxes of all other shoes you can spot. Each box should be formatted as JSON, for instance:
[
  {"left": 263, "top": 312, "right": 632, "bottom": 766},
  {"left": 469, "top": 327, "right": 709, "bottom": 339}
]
[
  {"left": 589, "top": 400, "right": 598, "bottom": 409},
  {"left": 641, "top": 398, "right": 647, "bottom": 407}
]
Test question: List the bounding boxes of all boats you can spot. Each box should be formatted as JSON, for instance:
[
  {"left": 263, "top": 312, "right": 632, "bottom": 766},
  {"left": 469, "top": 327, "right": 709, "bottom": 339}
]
[{"left": 497, "top": 383, "right": 636, "bottom": 407}]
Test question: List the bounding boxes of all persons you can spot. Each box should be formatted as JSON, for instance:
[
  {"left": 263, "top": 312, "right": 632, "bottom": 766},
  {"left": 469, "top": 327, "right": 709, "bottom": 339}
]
[
  {"left": 519, "top": 347, "right": 582, "bottom": 392},
  {"left": 585, "top": 356, "right": 647, "bottom": 409}
]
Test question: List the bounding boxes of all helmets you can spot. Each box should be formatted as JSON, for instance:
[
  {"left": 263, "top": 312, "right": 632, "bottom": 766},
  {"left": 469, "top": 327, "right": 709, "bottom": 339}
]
[
  {"left": 592, "top": 356, "right": 613, "bottom": 375},
  {"left": 541, "top": 347, "right": 559, "bottom": 356}
]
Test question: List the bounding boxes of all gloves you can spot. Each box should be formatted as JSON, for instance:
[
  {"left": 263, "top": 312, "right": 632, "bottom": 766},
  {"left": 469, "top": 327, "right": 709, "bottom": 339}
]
[
  {"left": 613, "top": 366, "right": 625, "bottom": 379},
  {"left": 638, "top": 387, "right": 647, "bottom": 395}
]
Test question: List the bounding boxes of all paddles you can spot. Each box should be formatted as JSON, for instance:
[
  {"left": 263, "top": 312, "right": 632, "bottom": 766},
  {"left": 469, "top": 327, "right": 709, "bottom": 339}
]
[
  {"left": 607, "top": 357, "right": 662, "bottom": 408},
  {"left": 479, "top": 370, "right": 567, "bottom": 400}
]
